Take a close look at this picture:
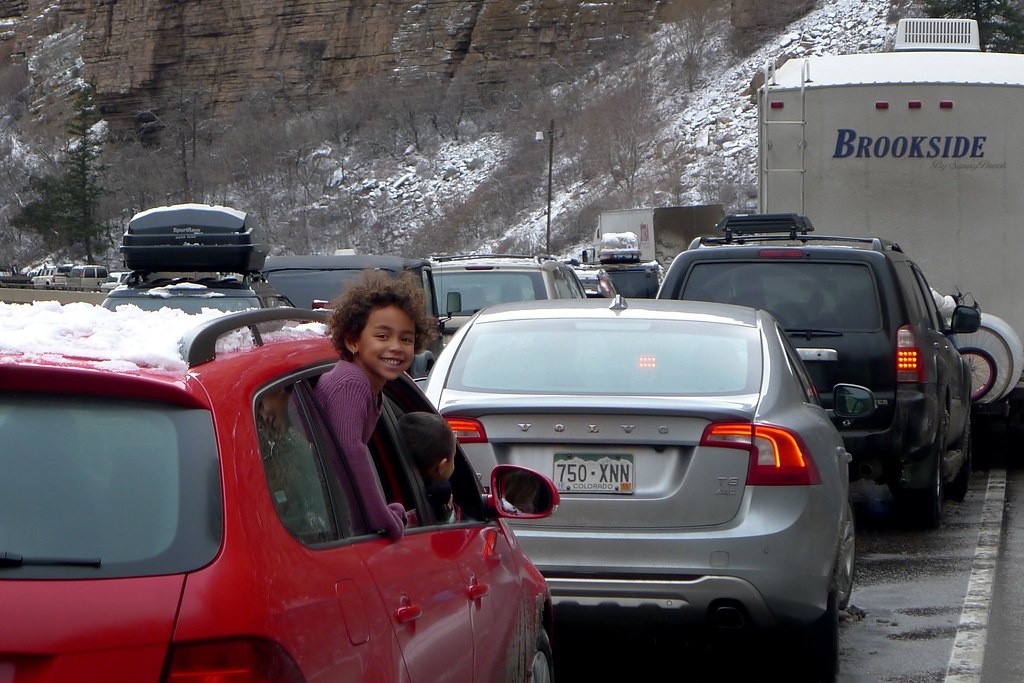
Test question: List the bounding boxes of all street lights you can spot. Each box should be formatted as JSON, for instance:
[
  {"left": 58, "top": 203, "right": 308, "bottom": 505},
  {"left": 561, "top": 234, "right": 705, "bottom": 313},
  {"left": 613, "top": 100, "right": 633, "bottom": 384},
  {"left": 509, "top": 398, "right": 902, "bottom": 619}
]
[{"left": 534, "top": 120, "right": 556, "bottom": 255}]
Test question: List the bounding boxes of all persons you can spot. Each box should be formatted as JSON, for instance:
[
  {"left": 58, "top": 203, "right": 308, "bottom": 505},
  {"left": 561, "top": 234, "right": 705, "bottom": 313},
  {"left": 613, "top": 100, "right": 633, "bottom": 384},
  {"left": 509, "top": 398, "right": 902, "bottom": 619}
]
[
  {"left": 827, "top": 263, "right": 882, "bottom": 331},
  {"left": 252, "top": 380, "right": 314, "bottom": 446},
  {"left": 306, "top": 272, "right": 473, "bottom": 541}
]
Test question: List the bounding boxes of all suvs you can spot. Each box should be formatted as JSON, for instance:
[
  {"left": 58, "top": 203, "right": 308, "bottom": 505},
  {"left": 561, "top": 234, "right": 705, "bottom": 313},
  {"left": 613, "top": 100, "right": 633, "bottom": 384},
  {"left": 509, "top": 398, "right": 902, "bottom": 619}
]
[
  {"left": 0, "top": 308, "right": 562, "bottom": 683},
  {"left": 260, "top": 256, "right": 462, "bottom": 380},
  {"left": 656, "top": 231, "right": 980, "bottom": 529}
]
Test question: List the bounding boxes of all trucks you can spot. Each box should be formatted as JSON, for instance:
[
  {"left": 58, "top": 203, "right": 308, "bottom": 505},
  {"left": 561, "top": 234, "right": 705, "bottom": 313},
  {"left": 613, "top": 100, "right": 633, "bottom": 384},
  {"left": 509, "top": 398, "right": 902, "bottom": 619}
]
[{"left": 758, "top": 17, "right": 1024, "bottom": 469}]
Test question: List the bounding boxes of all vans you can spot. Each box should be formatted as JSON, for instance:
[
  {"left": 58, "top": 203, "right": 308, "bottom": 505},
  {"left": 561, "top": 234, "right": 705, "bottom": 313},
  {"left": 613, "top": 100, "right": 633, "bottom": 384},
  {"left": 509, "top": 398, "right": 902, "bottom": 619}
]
[{"left": 65, "top": 265, "right": 108, "bottom": 292}]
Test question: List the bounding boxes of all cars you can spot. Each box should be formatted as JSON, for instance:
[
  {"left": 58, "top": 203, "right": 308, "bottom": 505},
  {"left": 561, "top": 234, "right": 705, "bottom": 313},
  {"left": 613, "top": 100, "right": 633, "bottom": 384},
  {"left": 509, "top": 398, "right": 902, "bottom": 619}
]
[
  {"left": 423, "top": 253, "right": 590, "bottom": 346},
  {"left": 419, "top": 295, "right": 878, "bottom": 683},
  {"left": 101, "top": 269, "right": 295, "bottom": 310},
  {"left": 559, "top": 259, "right": 619, "bottom": 299},
  {"left": 100, "top": 271, "right": 143, "bottom": 294},
  {"left": 0, "top": 248, "right": 81, "bottom": 290}
]
